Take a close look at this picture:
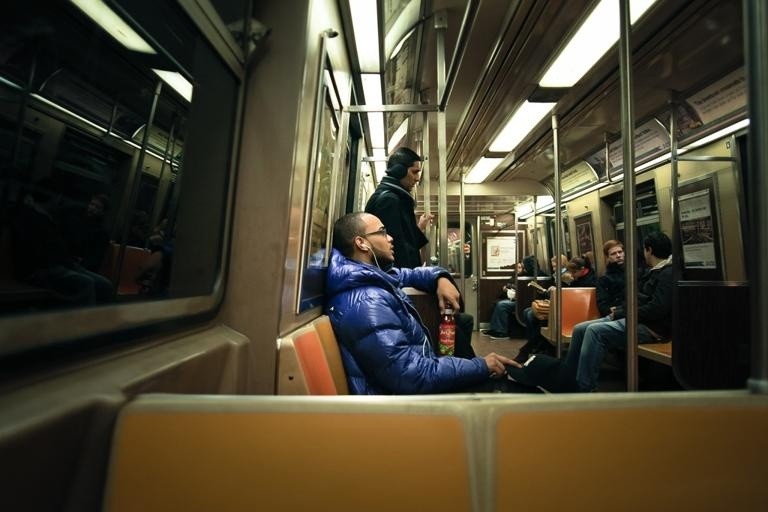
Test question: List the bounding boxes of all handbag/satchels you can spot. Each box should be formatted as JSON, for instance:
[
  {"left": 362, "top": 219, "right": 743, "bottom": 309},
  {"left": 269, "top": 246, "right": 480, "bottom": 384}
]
[
  {"left": 532, "top": 299, "right": 551, "bottom": 320},
  {"left": 506, "top": 288, "right": 516, "bottom": 299}
]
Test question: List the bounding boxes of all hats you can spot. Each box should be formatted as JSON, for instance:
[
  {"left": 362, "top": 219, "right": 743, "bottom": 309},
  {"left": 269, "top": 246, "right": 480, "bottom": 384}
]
[{"left": 505, "top": 353, "right": 569, "bottom": 387}]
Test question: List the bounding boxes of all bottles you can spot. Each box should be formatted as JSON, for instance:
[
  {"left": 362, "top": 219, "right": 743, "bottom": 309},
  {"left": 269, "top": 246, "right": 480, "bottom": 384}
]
[{"left": 438, "top": 309, "right": 456, "bottom": 357}]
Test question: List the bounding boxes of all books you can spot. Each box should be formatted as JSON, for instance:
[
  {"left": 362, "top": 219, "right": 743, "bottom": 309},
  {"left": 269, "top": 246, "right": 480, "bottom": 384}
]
[{"left": 527, "top": 279, "right": 546, "bottom": 294}]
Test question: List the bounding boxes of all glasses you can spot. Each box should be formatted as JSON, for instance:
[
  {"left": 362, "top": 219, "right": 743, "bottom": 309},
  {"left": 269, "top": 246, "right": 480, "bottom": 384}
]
[{"left": 362, "top": 226, "right": 387, "bottom": 238}]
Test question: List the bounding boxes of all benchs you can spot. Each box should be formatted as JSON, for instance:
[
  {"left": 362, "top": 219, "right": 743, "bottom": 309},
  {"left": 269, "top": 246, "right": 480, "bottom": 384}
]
[
  {"left": 111, "top": 244, "right": 145, "bottom": 295},
  {"left": 105, "top": 285, "right": 768, "bottom": 511}
]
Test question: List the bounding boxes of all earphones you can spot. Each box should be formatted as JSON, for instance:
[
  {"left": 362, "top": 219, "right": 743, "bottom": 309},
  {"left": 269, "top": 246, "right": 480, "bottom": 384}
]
[{"left": 362, "top": 244, "right": 369, "bottom": 248}]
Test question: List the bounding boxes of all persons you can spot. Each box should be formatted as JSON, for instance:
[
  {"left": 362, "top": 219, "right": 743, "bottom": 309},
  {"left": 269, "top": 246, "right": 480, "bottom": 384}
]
[
  {"left": 520, "top": 254, "right": 569, "bottom": 342},
  {"left": 512, "top": 250, "right": 596, "bottom": 366},
  {"left": 487, "top": 254, "right": 549, "bottom": 341},
  {"left": 594, "top": 239, "right": 644, "bottom": 320},
  {"left": 564, "top": 230, "right": 678, "bottom": 391},
  {"left": 360, "top": 145, "right": 438, "bottom": 268},
  {"left": 319, "top": 210, "right": 547, "bottom": 394},
  {"left": 434, "top": 229, "right": 470, "bottom": 272},
  {"left": 480, "top": 260, "right": 526, "bottom": 336}
]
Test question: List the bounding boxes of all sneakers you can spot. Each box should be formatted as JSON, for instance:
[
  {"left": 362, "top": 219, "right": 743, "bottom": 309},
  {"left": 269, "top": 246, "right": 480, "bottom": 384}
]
[{"left": 482, "top": 330, "right": 510, "bottom": 340}]
